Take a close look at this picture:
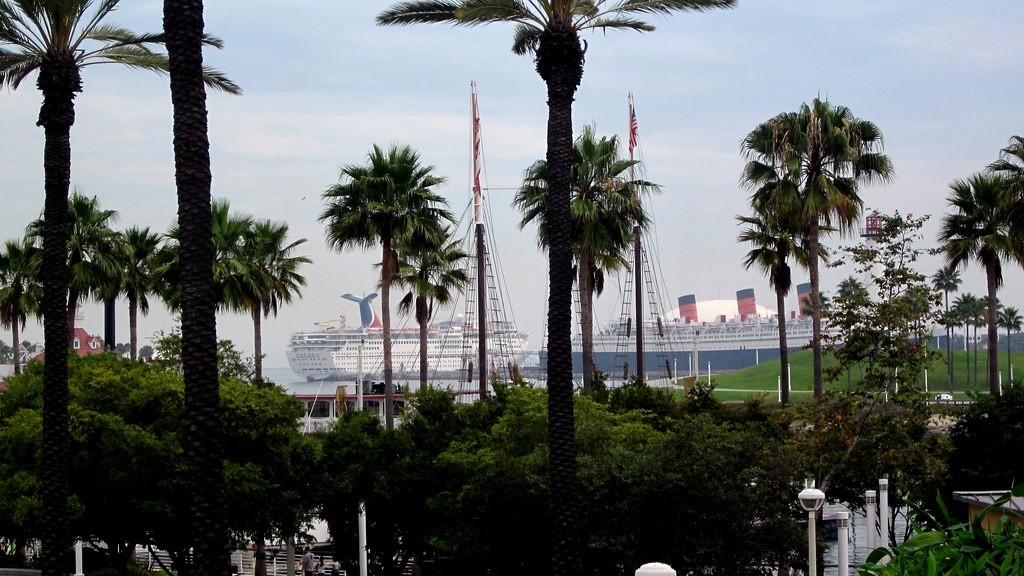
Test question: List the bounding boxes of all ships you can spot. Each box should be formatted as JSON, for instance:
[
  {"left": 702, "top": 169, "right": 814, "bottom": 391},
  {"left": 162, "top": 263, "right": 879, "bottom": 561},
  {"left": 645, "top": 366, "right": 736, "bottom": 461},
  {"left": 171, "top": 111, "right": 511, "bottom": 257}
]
[
  {"left": 537, "top": 283, "right": 847, "bottom": 375},
  {"left": 286, "top": 293, "right": 534, "bottom": 383}
]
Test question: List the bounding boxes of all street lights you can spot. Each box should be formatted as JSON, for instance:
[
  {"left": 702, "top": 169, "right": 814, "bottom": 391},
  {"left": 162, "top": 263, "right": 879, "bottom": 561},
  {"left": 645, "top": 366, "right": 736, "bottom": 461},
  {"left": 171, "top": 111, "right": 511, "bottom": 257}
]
[
  {"left": 836, "top": 512, "right": 848, "bottom": 576},
  {"left": 799, "top": 487, "right": 825, "bottom": 576},
  {"left": 879, "top": 478, "right": 888, "bottom": 548},
  {"left": 866, "top": 489, "right": 877, "bottom": 548}
]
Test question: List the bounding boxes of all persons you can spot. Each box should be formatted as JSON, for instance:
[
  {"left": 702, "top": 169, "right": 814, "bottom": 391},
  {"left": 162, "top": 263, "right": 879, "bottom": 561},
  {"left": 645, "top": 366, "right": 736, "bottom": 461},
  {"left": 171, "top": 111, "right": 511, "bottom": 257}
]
[{"left": 300, "top": 547, "right": 341, "bottom": 576}]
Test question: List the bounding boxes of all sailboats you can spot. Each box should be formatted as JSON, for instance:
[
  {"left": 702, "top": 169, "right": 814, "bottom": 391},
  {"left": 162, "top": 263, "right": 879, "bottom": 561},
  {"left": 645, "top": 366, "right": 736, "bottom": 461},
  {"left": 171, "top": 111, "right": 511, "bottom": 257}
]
[{"left": 288, "top": 79, "right": 533, "bottom": 435}]
